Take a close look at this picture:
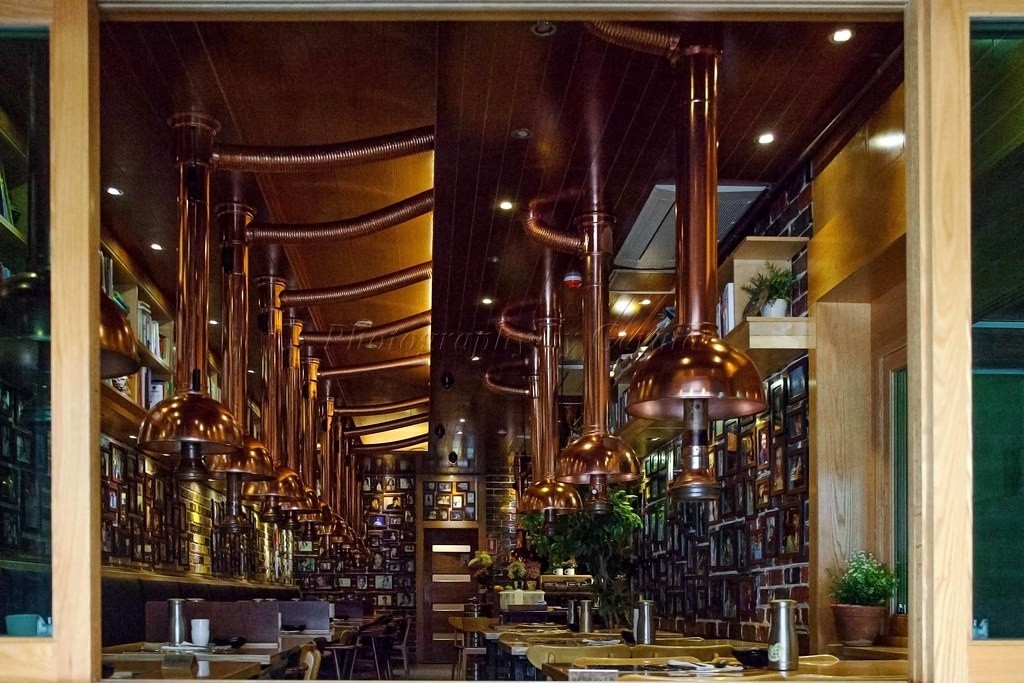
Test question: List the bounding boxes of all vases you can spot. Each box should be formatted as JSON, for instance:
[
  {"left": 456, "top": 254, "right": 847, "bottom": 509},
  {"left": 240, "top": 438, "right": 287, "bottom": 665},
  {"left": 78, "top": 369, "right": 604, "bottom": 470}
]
[
  {"left": 514, "top": 581, "right": 524, "bottom": 589},
  {"left": 526, "top": 581, "right": 537, "bottom": 591},
  {"left": 830, "top": 603, "right": 888, "bottom": 648},
  {"left": 478, "top": 585, "right": 488, "bottom": 594}
]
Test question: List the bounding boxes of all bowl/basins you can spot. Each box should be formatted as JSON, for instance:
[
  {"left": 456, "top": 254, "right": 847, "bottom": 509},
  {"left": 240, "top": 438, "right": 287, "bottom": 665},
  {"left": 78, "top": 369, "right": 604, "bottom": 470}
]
[
  {"left": 621, "top": 631, "right": 634, "bottom": 642},
  {"left": 730, "top": 650, "right": 766, "bottom": 667},
  {"left": 213, "top": 637, "right": 247, "bottom": 649}
]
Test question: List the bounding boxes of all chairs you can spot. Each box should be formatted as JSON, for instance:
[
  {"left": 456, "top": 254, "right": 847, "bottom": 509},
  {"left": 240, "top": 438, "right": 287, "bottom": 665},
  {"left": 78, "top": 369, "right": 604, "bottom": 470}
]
[
  {"left": 448, "top": 617, "right": 908, "bottom": 681},
  {"left": 258, "top": 612, "right": 415, "bottom": 681}
]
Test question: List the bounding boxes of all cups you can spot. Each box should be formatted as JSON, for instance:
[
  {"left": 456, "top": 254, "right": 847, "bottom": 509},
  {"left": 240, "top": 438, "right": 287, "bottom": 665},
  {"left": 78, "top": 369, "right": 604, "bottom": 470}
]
[
  {"left": 564, "top": 569, "right": 574, "bottom": 575},
  {"left": 5, "top": 614, "right": 39, "bottom": 636},
  {"left": 553, "top": 568, "right": 563, "bottom": 575},
  {"left": 167, "top": 598, "right": 188, "bottom": 645},
  {"left": 329, "top": 604, "right": 334, "bottom": 617},
  {"left": 191, "top": 619, "right": 209, "bottom": 646}
]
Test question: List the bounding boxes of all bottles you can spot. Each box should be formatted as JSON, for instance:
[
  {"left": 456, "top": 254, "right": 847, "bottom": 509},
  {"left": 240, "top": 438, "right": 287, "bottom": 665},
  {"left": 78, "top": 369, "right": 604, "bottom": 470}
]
[
  {"left": 637, "top": 600, "right": 655, "bottom": 645},
  {"left": 567, "top": 600, "right": 579, "bottom": 632},
  {"left": 580, "top": 600, "right": 593, "bottom": 633},
  {"left": 768, "top": 599, "right": 798, "bottom": 671}
]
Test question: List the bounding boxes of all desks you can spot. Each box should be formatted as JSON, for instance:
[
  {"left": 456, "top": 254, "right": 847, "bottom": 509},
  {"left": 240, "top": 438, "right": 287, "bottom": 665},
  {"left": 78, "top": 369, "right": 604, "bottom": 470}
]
[
  {"left": 101, "top": 642, "right": 299, "bottom": 665},
  {"left": 281, "top": 629, "right": 342, "bottom": 642},
  {"left": 542, "top": 659, "right": 908, "bottom": 681},
  {"left": 334, "top": 618, "right": 380, "bottom": 629},
  {"left": 479, "top": 629, "right": 684, "bottom": 680},
  {"left": 101, "top": 660, "right": 261, "bottom": 680},
  {"left": 505, "top": 638, "right": 768, "bottom": 681}
]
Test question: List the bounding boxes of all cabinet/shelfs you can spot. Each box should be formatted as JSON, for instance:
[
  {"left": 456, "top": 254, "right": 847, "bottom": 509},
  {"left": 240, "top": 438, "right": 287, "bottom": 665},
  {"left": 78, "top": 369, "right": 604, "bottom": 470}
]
[
  {"left": 614, "top": 316, "right": 810, "bottom": 458},
  {"left": 0, "top": 110, "right": 262, "bottom": 511}
]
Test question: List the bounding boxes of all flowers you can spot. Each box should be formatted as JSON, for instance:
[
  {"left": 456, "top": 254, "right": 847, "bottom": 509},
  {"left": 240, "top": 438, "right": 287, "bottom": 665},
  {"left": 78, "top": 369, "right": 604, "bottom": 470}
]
[
  {"left": 506, "top": 561, "right": 541, "bottom": 581},
  {"left": 468, "top": 551, "right": 494, "bottom": 585},
  {"left": 828, "top": 549, "right": 900, "bottom": 607}
]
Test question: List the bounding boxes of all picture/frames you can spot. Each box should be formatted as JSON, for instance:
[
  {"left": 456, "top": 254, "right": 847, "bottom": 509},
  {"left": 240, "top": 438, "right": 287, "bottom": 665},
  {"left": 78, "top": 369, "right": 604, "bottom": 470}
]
[
  {"left": 626, "top": 356, "right": 808, "bottom": 619},
  {"left": 292, "top": 475, "right": 418, "bottom": 608},
  {"left": 424, "top": 481, "right": 476, "bottom": 521},
  {"left": 0, "top": 382, "right": 190, "bottom": 567}
]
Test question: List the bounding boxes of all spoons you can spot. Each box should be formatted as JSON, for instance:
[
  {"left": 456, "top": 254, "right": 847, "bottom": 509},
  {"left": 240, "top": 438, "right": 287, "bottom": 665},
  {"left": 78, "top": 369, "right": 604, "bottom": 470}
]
[{"left": 708, "top": 660, "right": 726, "bottom": 668}]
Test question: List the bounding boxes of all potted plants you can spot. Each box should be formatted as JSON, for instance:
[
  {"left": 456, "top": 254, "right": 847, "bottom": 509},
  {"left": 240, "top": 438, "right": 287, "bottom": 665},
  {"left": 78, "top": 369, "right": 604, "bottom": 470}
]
[{"left": 739, "top": 261, "right": 801, "bottom": 317}]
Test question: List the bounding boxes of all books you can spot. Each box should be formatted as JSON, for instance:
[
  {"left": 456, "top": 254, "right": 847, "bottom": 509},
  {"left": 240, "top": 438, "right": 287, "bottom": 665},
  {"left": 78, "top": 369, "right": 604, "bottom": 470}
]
[
  {"left": 135, "top": 358, "right": 175, "bottom": 416},
  {"left": 608, "top": 386, "right": 635, "bottom": 435},
  {"left": 138, "top": 306, "right": 175, "bottom": 368},
  {"left": 97, "top": 242, "right": 118, "bottom": 296}
]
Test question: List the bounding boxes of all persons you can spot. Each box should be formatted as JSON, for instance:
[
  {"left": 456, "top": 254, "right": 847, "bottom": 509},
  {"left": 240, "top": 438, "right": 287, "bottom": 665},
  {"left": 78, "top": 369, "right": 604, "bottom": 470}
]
[
  {"left": 425, "top": 482, "right": 477, "bottom": 521},
  {"left": 298, "top": 456, "right": 414, "bottom": 607},
  {"left": 100, "top": 439, "right": 187, "bottom": 570},
  {"left": 637, "top": 362, "right": 806, "bottom": 622}
]
